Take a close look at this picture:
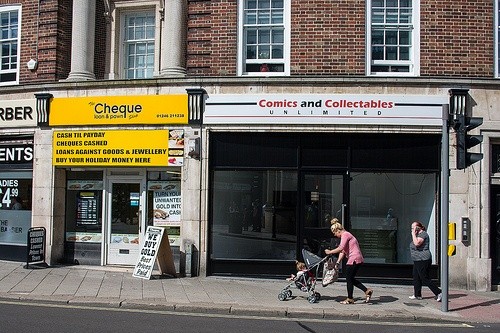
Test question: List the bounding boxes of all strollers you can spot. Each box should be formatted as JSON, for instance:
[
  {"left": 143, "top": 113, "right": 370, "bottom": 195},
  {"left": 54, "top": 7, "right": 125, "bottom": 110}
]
[{"left": 278, "top": 246, "right": 333, "bottom": 304}]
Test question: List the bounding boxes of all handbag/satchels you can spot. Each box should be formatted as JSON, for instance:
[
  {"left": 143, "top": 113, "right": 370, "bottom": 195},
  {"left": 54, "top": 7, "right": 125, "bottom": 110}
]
[{"left": 322, "top": 257, "right": 338, "bottom": 287}]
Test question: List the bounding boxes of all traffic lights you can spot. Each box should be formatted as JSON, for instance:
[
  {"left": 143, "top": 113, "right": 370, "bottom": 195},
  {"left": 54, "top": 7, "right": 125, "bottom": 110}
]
[{"left": 448, "top": 88, "right": 484, "bottom": 169}]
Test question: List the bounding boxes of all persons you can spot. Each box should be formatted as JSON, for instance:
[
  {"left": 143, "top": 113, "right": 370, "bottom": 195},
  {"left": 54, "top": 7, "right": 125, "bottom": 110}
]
[
  {"left": 303, "top": 200, "right": 342, "bottom": 258},
  {"left": 111, "top": 188, "right": 136, "bottom": 224},
  {"left": 408, "top": 220, "right": 443, "bottom": 301},
  {"left": 290, "top": 259, "right": 309, "bottom": 279},
  {"left": 324, "top": 217, "right": 373, "bottom": 305},
  {"left": 229, "top": 198, "right": 264, "bottom": 235},
  {"left": 10, "top": 197, "right": 24, "bottom": 210}
]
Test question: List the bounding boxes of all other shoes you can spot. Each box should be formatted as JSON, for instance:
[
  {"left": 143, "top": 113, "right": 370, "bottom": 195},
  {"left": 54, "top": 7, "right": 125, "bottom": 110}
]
[
  {"left": 437, "top": 293, "right": 442, "bottom": 301},
  {"left": 340, "top": 297, "right": 354, "bottom": 304},
  {"left": 364, "top": 288, "right": 373, "bottom": 302},
  {"left": 409, "top": 294, "right": 423, "bottom": 299}
]
